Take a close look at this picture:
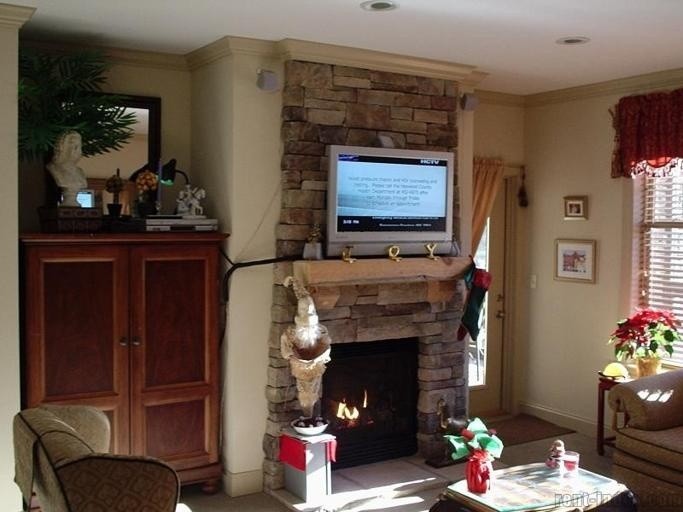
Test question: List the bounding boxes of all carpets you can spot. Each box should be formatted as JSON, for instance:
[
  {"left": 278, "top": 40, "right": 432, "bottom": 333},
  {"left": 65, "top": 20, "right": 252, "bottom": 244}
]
[{"left": 476, "top": 411, "right": 578, "bottom": 450}]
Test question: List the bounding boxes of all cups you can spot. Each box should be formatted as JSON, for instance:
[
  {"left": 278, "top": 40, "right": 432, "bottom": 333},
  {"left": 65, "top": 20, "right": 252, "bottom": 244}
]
[
  {"left": 558, "top": 451, "right": 580, "bottom": 480},
  {"left": 107, "top": 203, "right": 121, "bottom": 217},
  {"left": 625, "top": 364, "right": 637, "bottom": 379}
]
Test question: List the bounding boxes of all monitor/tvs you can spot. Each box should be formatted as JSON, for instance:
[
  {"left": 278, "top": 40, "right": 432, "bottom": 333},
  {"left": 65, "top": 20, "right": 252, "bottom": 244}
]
[
  {"left": 61, "top": 189, "right": 95, "bottom": 208},
  {"left": 325, "top": 144, "right": 454, "bottom": 257}
]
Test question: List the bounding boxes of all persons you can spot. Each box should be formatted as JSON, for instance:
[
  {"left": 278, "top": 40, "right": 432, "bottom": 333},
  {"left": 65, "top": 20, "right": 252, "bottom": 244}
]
[{"left": 48, "top": 130, "right": 87, "bottom": 187}]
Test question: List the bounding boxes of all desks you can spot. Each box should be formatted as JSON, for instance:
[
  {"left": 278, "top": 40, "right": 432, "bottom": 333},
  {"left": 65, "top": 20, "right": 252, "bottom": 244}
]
[{"left": 595, "top": 375, "right": 636, "bottom": 454}]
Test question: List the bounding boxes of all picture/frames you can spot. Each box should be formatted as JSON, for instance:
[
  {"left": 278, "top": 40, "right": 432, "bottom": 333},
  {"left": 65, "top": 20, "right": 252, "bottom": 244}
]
[
  {"left": 562, "top": 193, "right": 589, "bottom": 222},
  {"left": 549, "top": 237, "right": 599, "bottom": 286}
]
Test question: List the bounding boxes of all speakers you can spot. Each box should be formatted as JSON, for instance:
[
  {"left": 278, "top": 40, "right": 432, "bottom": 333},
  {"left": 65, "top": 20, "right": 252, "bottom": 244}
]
[
  {"left": 259, "top": 71, "right": 277, "bottom": 91},
  {"left": 460, "top": 93, "right": 476, "bottom": 111}
]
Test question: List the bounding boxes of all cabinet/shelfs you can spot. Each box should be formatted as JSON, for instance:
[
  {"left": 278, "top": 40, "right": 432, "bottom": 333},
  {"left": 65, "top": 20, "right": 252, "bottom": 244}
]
[{"left": 14, "top": 227, "right": 230, "bottom": 491}]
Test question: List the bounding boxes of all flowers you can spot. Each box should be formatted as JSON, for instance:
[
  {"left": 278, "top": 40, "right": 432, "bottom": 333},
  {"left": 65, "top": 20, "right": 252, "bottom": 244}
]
[
  {"left": 103, "top": 172, "right": 126, "bottom": 192},
  {"left": 136, "top": 168, "right": 157, "bottom": 189},
  {"left": 604, "top": 306, "right": 682, "bottom": 363}
]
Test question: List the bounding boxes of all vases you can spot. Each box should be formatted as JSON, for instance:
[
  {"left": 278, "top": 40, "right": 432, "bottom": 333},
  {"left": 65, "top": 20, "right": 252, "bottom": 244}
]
[
  {"left": 107, "top": 200, "right": 123, "bottom": 214},
  {"left": 634, "top": 355, "right": 657, "bottom": 380}
]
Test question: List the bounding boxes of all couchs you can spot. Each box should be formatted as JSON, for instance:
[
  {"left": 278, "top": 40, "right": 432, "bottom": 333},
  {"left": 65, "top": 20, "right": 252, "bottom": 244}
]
[
  {"left": 604, "top": 368, "right": 683, "bottom": 510},
  {"left": 11, "top": 399, "right": 192, "bottom": 512}
]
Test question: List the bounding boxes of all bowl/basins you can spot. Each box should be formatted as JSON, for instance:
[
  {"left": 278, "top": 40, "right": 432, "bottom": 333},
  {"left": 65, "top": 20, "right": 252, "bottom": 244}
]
[{"left": 290, "top": 417, "right": 331, "bottom": 436}]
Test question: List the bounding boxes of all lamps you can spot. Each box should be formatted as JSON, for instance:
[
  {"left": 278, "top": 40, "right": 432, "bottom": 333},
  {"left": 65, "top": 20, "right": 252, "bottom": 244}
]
[{"left": 158, "top": 157, "right": 191, "bottom": 216}]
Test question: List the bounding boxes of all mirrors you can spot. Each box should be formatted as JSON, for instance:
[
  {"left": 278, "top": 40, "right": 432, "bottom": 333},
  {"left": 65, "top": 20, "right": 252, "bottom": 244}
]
[{"left": 42, "top": 88, "right": 162, "bottom": 221}]
